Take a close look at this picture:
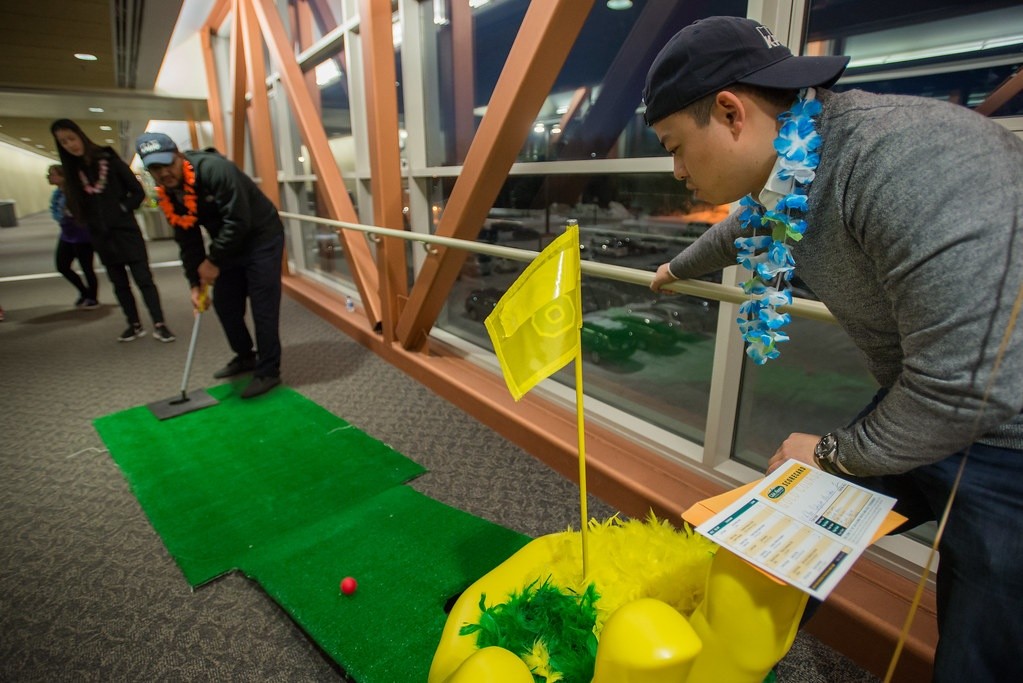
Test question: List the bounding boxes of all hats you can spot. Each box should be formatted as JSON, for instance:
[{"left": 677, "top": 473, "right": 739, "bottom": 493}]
[
  {"left": 641, "top": 15, "right": 850, "bottom": 128},
  {"left": 135, "top": 133, "right": 178, "bottom": 169}
]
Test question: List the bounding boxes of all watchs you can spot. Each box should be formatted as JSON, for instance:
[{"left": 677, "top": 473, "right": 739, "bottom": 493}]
[{"left": 813, "top": 432, "right": 863, "bottom": 483}]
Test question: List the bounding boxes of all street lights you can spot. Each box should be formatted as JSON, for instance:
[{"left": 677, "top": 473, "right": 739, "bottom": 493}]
[{"left": 535, "top": 120, "right": 562, "bottom": 235}]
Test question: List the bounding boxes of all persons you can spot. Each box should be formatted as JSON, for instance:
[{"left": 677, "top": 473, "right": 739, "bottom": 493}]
[
  {"left": 46, "top": 165, "right": 102, "bottom": 311},
  {"left": 50, "top": 119, "right": 176, "bottom": 344},
  {"left": 643, "top": 17, "right": 1023, "bottom": 683},
  {"left": 135, "top": 132, "right": 286, "bottom": 399}
]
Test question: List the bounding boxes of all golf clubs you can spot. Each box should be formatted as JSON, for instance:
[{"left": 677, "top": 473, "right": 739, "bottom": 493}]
[{"left": 168, "top": 283, "right": 209, "bottom": 407}]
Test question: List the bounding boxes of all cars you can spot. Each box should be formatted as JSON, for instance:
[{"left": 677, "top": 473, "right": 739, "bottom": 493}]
[
  {"left": 590, "top": 232, "right": 668, "bottom": 258},
  {"left": 466, "top": 287, "right": 507, "bottom": 320},
  {"left": 582, "top": 293, "right": 719, "bottom": 364}
]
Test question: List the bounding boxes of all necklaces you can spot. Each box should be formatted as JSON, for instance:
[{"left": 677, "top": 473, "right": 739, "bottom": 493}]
[
  {"left": 733, "top": 97, "right": 822, "bottom": 364},
  {"left": 78, "top": 160, "right": 107, "bottom": 193},
  {"left": 50, "top": 190, "right": 66, "bottom": 221},
  {"left": 155, "top": 158, "right": 197, "bottom": 230}
]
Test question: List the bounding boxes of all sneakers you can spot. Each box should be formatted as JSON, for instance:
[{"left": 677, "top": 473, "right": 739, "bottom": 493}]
[
  {"left": 117, "top": 325, "right": 145, "bottom": 341},
  {"left": 152, "top": 325, "right": 176, "bottom": 343}
]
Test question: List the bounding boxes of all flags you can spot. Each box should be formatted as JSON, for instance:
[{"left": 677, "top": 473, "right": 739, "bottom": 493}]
[{"left": 483, "top": 227, "right": 584, "bottom": 403}]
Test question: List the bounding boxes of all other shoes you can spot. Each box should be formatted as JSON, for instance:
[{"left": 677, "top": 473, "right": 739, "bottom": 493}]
[
  {"left": 74, "top": 293, "right": 88, "bottom": 307},
  {"left": 86, "top": 300, "right": 99, "bottom": 309},
  {"left": 213, "top": 352, "right": 259, "bottom": 378},
  {"left": 240, "top": 376, "right": 279, "bottom": 397}
]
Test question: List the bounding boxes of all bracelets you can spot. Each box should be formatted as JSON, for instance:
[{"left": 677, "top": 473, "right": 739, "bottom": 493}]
[{"left": 668, "top": 263, "right": 680, "bottom": 280}]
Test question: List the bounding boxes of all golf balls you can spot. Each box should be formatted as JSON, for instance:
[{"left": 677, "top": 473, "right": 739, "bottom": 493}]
[{"left": 340, "top": 576, "right": 358, "bottom": 595}]
